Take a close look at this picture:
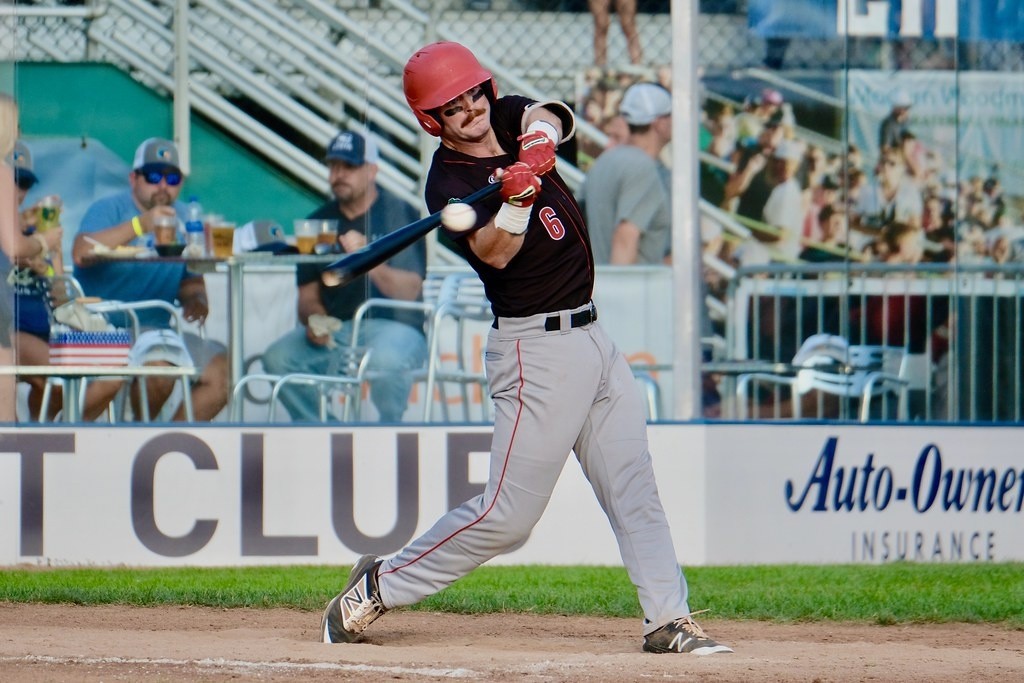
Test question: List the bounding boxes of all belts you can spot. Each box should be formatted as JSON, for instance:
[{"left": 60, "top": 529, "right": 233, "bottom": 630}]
[{"left": 491, "top": 298, "right": 598, "bottom": 332}]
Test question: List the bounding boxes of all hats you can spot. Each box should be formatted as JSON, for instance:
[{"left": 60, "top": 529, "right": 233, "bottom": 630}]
[
  {"left": 326, "top": 131, "right": 380, "bottom": 166},
  {"left": 133, "top": 138, "right": 185, "bottom": 177},
  {"left": 12, "top": 141, "right": 38, "bottom": 186},
  {"left": 618, "top": 81, "right": 672, "bottom": 127}
]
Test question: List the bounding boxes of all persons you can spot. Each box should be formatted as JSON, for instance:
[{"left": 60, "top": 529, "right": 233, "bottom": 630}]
[
  {"left": 0, "top": 94, "right": 131, "bottom": 422},
  {"left": 320, "top": 42, "right": 734, "bottom": 656},
  {"left": 563, "top": 0, "right": 1023, "bottom": 418},
  {"left": 258, "top": 131, "right": 427, "bottom": 421},
  {"left": 72, "top": 137, "right": 234, "bottom": 421}
]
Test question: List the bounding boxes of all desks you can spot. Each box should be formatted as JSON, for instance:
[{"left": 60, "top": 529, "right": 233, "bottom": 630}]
[{"left": 84, "top": 254, "right": 351, "bottom": 421}]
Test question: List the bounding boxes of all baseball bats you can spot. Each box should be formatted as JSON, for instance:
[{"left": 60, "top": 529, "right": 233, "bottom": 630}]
[{"left": 323, "top": 181, "right": 502, "bottom": 287}]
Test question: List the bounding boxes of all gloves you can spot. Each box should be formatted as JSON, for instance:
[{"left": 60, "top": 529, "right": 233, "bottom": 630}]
[
  {"left": 488, "top": 161, "right": 542, "bottom": 208},
  {"left": 515, "top": 131, "right": 557, "bottom": 177}
]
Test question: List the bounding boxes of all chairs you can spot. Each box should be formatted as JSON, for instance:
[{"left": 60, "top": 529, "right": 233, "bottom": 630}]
[{"left": 0, "top": 264, "right": 907, "bottom": 424}]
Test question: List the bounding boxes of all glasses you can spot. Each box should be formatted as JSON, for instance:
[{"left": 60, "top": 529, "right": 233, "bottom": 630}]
[
  {"left": 135, "top": 168, "right": 182, "bottom": 185},
  {"left": 14, "top": 176, "right": 35, "bottom": 191}
]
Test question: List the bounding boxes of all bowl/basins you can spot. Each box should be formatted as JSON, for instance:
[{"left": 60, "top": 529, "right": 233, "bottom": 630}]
[{"left": 155, "top": 244, "right": 186, "bottom": 257}]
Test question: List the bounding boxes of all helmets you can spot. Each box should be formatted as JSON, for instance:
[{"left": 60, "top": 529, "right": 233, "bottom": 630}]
[{"left": 402, "top": 42, "right": 500, "bottom": 137}]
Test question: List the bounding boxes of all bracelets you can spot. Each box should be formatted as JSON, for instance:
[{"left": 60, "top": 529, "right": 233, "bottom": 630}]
[
  {"left": 32, "top": 232, "right": 50, "bottom": 256},
  {"left": 131, "top": 216, "right": 144, "bottom": 238}
]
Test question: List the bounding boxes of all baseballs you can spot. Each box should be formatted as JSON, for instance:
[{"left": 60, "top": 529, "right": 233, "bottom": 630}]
[{"left": 440, "top": 202, "right": 478, "bottom": 231}]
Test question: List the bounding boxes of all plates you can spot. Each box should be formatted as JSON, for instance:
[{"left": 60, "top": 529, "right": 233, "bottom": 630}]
[{"left": 89, "top": 249, "right": 145, "bottom": 259}]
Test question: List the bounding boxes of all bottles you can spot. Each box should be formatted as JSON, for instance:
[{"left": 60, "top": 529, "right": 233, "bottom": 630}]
[
  {"left": 22, "top": 225, "right": 56, "bottom": 279},
  {"left": 38, "top": 197, "right": 61, "bottom": 232},
  {"left": 185, "top": 197, "right": 205, "bottom": 257}
]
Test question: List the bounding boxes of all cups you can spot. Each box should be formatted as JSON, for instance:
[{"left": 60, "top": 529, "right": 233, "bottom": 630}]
[
  {"left": 293, "top": 219, "right": 320, "bottom": 254},
  {"left": 200, "top": 215, "right": 221, "bottom": 254},
  {"left": 211, "top": 223, "right": 235, "bottom": 258},
  {"left": 156, "top": 215, "right": 177, "bottom": 245},
  {"left": 313, "top": 219, "right": 338, "bottom": 254},
  {"left": 320, "top": 381, "right": 362, "bottom": 424}
]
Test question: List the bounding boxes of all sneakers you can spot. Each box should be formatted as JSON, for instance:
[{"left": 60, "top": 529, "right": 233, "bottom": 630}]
[
  {"left": 643, "top": 613, "right": 736, "bottom": 655},
  {"left": 319, "top": 552, "right": 389, "bottom": 644}
]
